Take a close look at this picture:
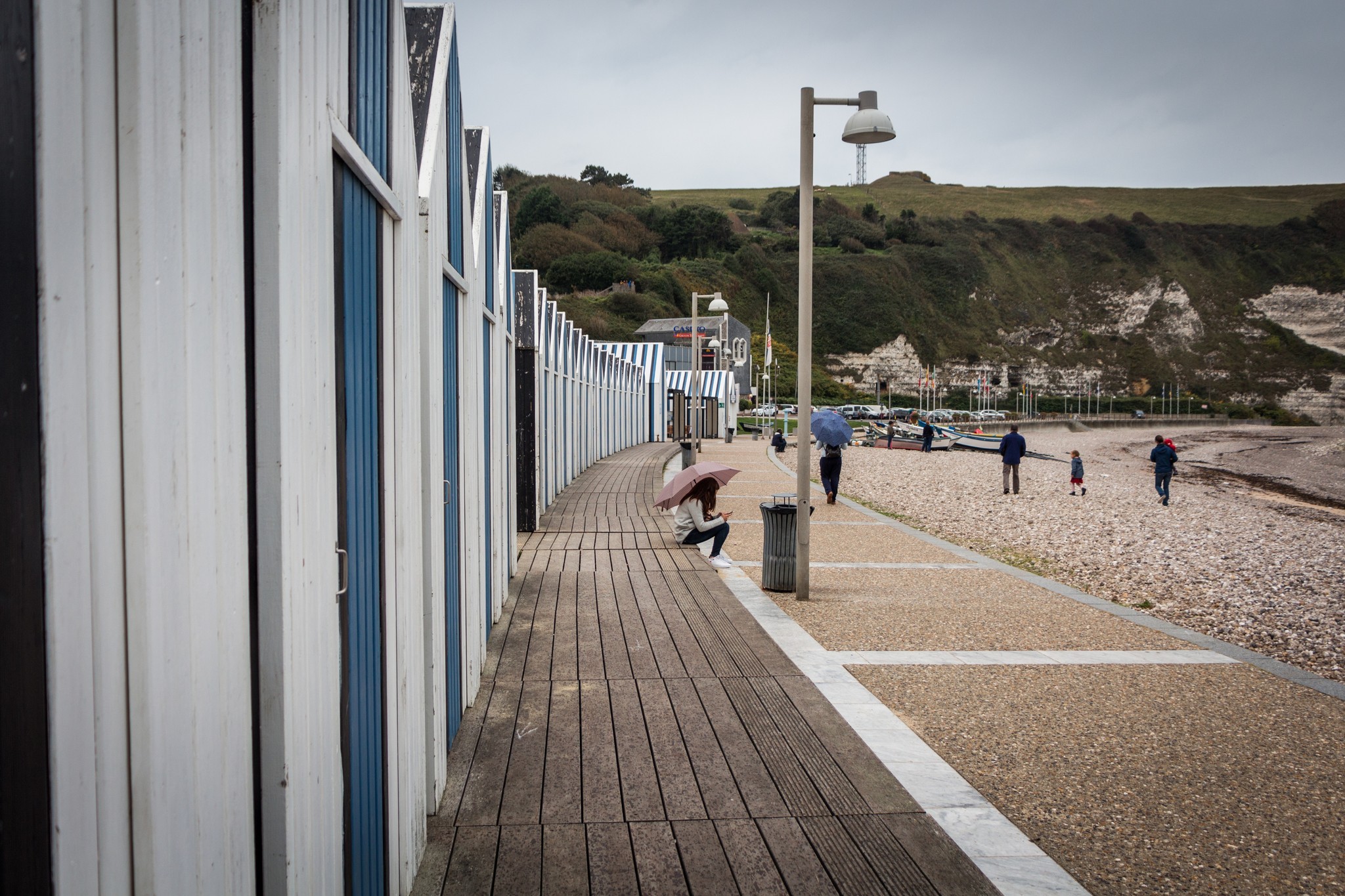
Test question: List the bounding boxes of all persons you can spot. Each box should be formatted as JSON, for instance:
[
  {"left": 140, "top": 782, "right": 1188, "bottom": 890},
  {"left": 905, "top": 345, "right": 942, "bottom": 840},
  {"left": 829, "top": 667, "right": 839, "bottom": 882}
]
[
  {"left": 673, "top": 477, "right": 733, "bottom": 568},
  {"left": 1069, "top": 451, "right": 1086, "bottom": 496},
  {"left": 1000, "top": 425, "right": 1026, "bottom": 495},
  {"left": 770, "top": 428, "right": 786, "bottom": 453},
  {"left": 920, "top": 421, "right": 934, "bottom": 453},
  {"left": 887, "top": 421, "right": 896, "bottom": 450},
  {"left": 1150, "top": 435, "right": 1178, "bottom": 506},
  {"left": 815, "top": 440, "right": 848, "bottom": 505},
  {"left": 1164, "top": 439, "right": 1179, "bottom": 476}
]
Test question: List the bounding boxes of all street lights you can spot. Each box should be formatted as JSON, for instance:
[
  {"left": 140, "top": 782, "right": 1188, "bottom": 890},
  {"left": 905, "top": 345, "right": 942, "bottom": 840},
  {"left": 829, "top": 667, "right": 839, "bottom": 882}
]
[
  {"left": 1188, "top": 397, "right": 1193, "bottom": 415},
  {"left": 795, "top": 85, "right": 896, "bottom": 593},
  {"left": 995, "top": 391, "right": 1002, "bottom": 411},
  {"left": 1016, "top": 392, "right": 1023, "bottom": 414},
  {"left": 1109, "top": 395, "right": 1116, "bottom": 419},
  {"left": 1064, "top": 394, "right": 1070, "bottom": 415},
  {"left": 940, "top": 387, "right": 977, "bottom": 412},
  {"left": 889, "top": 385, "right": 894, "bottom": 420},
  {"left": 756, "top": 372, "right": 771, "bottom": 422},
  {"left": 691, "top": 292, "right": 729, "bottom": 467},
  {"left": 698, "top": 334, "right": 744, "bottom": 454},
  {"left": 1035, "top": 393, "right": 1042, "bottom": 420},
  {"left": 1150, "top": 396, "right": 1157, "bottom": 419}
]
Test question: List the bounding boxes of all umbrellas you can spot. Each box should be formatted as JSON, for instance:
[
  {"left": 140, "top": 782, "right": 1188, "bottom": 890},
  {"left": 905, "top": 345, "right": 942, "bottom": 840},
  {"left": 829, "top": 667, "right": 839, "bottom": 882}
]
[
  {"left": 810, "top": 410, "right": 854, "bottom": 447},
  {"left": 652, "top": 462, "right": 743, "bottom": 512}
]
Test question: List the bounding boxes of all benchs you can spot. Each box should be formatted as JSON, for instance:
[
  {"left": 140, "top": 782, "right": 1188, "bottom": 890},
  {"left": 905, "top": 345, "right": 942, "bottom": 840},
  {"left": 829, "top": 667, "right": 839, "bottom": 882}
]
[{"left": 667, "top": 426, "right": 690, "bottom": 438}]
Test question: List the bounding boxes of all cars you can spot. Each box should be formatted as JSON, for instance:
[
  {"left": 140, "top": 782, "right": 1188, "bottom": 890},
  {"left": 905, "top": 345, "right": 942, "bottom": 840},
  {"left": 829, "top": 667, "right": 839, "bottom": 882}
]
[
  {"left": 793, "top": 405, "right": 821, "bottom": 415},
  {"left": 751, "top": 405, "right": 776, "bottom": 417},
  {"left": 845, "top": 404, "right": 1012, "bottom": 424},
  {"left": 1028, "top": 411, "right": 1040, "bottom": 417}
]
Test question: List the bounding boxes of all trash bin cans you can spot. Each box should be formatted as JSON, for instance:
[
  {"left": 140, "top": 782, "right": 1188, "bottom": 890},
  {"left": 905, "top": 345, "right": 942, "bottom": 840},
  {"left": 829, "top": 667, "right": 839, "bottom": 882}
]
[
  {"left": 1063, "top": 414, "right": 1069, "bottom": 420},
  {"left": 751, "top": 428, "right": 759, "bottom": 440},
  {"left": 1211, "top": 414, "right": 1215, "bottom": 418},
  {"left": 725, "top": 427, "right": 734, "bottom": 443},
  {"left": 680, "top": 442, "right": 699, "bottom": 471},
  {"left": 759, "top": 493, "right": 814, "bottom": 593},
  {"left": 989, "top": 416, "right": 992, "bottom": 421}
]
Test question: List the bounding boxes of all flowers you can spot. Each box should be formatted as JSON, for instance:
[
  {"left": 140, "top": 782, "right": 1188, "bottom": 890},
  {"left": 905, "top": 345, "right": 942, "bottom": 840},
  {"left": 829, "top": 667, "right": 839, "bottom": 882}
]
[
  {"left": 1050, "top": 412, "right": 1058, "bottom": 417},
  {"left": 909, "top": 410, "right": 920, "bottom": 420},
  {"left": 1040, "top": 412, "right": 1047, "bottom": 416}
]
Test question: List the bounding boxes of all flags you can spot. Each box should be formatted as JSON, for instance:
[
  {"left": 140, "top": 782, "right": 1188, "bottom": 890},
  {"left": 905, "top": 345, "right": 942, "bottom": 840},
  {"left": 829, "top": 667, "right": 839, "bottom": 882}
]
[
  {"left": 986, "top": 374, "right": 989, "bottom": 393},
  {"left": 932, "top": 368, "right": 934, "bottom": 389},
  {"left": 1162, "top": 386, "right": 1179, "bottom": 398},
  {"left": 983, "top": 374, "right": 985, "bottom": 391},
  {"left": 925, "top": 366, "right": 928, "bottom": 387},
  {"left": 978, "top": 372, "right": 980, "bottom": 391},
  {"left": 918, "top": 365, "right": 921, "bottom": 388},
  {"left": 1076, "top": 383, "right": 1100, "bottom": 397},
  {"left": 766, "top": 326, "right": 772, "bottom": 366},
  {"left": 1021, "top": 381, "right": 1033, "bottom": 398}
]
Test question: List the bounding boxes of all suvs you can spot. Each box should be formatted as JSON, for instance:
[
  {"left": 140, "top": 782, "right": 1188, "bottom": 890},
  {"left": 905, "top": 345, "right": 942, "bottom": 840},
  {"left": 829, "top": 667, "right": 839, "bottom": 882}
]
[
  {"left": 760, "top": 403, "right": 779, "bottom": 414},
  {"left": 818, "top": 407, "right": 848, "bottom": 421}
]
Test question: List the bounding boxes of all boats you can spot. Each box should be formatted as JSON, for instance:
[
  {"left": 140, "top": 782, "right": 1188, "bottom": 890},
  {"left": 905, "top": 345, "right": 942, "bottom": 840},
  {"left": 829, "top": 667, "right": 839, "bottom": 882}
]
[
  {"left": 861, "top": 419, "right": 1011, "bottom": 453},
  {"left": 872, "top": 434, "right": 929, "bottom": 452},
  {"left": 738, "top": 422, "right": 774, "bottom": 432}
]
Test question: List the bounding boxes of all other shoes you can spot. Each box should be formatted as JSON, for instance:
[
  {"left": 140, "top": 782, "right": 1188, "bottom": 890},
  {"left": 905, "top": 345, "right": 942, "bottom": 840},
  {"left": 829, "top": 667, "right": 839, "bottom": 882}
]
[
  {"left": 888, "top": 447, "right": 891, "bottom": 449},
  {"left": 1158, "top": 495, "right": 1166, "bottom": 503},
  {"left": 1004, "top": 489, "right": 1009, "bottom": 494},
  {"left": 1014, "top": 492, "right": 1019, "bottom": 494},
  {"left": 832, "top": 501, "right": 835, "bottom": 504},
  {"left": 921, "top": 450, "right": 931, "bottom": 453},
  {"left": 1081, "top": 487, "right": 1086, "bottom": 496},
  {"left": 1069, "top": 491, "right": 1075, "bottom": 495},
  {"left": 827, "top": 492, "right": 833, "bottom": 504}
]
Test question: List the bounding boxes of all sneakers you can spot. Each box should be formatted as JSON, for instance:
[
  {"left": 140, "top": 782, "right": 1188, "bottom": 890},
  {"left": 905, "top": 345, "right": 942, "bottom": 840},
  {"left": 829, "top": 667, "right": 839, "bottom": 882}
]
[
  {"left": 708, "top": 555, "right": 731, "bottom": 568},
  {"left": 718, "top": 554, "right": 732, "bottom": 563}
]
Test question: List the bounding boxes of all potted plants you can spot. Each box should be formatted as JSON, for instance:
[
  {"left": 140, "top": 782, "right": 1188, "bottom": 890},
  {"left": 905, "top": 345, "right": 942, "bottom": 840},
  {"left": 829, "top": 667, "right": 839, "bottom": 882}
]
[
  {"left": 952, "top": 412, "right": 961, "bottom": 423},
  {"left": 1004, "top": 412, "right": 1017, "bottom": 421},
  {"left": 960, "top": 412, "right": 969, "bottom": 422}
]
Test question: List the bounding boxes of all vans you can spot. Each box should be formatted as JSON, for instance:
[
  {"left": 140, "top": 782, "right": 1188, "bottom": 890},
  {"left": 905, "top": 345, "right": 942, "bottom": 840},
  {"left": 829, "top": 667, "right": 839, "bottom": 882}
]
[
  {"left": 1130, "top": 409, "right": 1145, "bottom": 419},
  {"left": 780, "top": 404, "right": 795, "bottom": 415},
  {"left": 837, "top": 406, "right": 858, "bottom": 419}
]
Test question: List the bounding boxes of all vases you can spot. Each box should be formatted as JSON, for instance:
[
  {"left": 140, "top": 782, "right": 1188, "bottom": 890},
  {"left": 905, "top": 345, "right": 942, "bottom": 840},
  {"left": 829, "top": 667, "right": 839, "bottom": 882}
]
[
  {"left": 1041, "top": 415, "right": 1046, "bottom": 420},
  {"left": 911, "top": 417, "right": 918, "bottom": 424},
  {"left": 1053, "top": 416, "right": 1056, "bottom": 419}
]
